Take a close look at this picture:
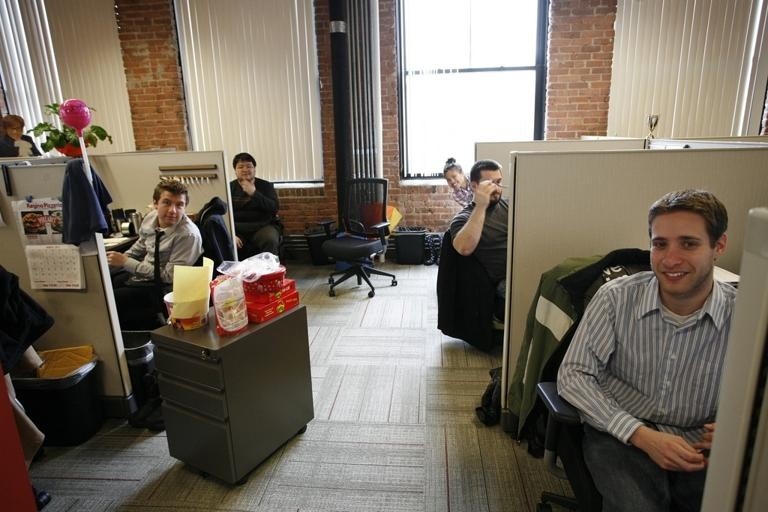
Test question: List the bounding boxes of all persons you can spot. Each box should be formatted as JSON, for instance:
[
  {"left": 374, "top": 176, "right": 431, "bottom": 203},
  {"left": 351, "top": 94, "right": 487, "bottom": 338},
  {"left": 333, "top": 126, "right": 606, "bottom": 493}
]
[
  {"left": 449, "top": 161, "right": 508, "bottom": 325},
  {"left": 0, "top": 113, "right": 43, "bottom": 157},
  {"left": 443, "top": 157, "right": 475, "bottom": 209},
  {"left": 552, "top": 187, "right": 742, "bottom": 512},
  {"left": 230, "top": 153, "right": 283, "bottom": 261},
  {"left": 106, "top": 179, "right": 205, "bottom": 321}
]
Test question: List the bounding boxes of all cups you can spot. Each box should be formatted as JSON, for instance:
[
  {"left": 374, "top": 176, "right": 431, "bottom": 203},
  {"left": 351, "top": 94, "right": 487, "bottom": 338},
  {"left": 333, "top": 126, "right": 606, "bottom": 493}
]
[
  {"left": 163, "top": 290, "right": 211, "bottom": 333},
  {"left": 120, "top": 222, "right": 134, "bottom": 236},
  {"left": 132, "top": 212, "right": 141, "bottom": 235}
]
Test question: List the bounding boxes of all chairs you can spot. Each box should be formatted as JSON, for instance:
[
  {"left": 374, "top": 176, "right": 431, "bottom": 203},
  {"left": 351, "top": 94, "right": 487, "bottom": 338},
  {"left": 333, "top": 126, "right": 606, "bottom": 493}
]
[
  {"left": 532, "top": 267, "right": 652, "bottom": 512},
  {"left": 320, "top": 177, "right": 398, "bottom": 298}
]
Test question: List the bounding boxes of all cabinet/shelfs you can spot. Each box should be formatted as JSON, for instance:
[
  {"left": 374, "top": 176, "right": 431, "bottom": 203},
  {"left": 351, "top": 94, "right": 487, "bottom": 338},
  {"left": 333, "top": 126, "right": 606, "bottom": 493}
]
[{"left": 151, "top": 304, "right": 316, "bottom": 487}]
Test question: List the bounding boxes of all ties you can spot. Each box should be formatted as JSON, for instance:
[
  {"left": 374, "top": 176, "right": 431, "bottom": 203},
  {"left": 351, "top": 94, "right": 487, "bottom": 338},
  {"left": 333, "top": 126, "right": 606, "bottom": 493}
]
[{"left": 154, "top": 229, "right": 165, "bottom": 282}]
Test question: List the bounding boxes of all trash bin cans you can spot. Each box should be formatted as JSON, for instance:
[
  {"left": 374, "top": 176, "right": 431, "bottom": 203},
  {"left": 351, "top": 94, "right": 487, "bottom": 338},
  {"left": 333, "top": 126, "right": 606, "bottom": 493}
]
[
  {"left": 10, "top": 353, "right": 100, "bottom": 455},
  {"left": 123, "top": 338, "right": 161, "bottom": 409},
  {"left": 393, "top": 226, "right": 426, "bottom": 265}
]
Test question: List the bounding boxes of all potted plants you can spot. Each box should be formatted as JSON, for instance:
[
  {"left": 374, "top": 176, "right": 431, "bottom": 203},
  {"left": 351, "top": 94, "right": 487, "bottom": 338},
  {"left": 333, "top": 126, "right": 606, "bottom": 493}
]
[{"left": 26, "top": 101, "right": 115, "bottom": 159}]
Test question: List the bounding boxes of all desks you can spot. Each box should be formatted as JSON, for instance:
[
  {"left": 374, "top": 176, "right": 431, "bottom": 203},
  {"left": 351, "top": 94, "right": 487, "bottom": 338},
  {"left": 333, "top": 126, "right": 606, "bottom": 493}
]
[{"left": 102, "top": 229, "right": 141, "bottom": 253}]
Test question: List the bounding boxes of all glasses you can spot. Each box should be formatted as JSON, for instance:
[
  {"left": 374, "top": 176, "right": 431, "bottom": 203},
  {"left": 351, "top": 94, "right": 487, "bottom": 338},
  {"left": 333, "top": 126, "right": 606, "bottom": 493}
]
[
  {"left": 236, "top": 165, "right": 253, "bottom": 170},
  {"left": 7, "top": 127, "right": 23, "bottom": 132}
]
